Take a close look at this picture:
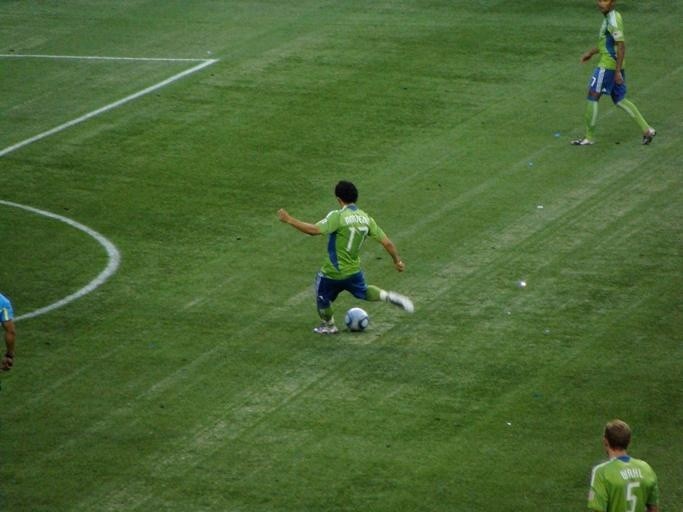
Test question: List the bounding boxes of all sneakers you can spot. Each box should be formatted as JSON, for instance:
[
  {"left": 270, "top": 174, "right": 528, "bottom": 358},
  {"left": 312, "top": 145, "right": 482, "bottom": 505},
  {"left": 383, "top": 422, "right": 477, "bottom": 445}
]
[
  {"left": 313, "top": 326, "right": 339, "bottom": 334},
  {"left": 389, "top": 292, "right": 414, "bottom": 313},
  {"left": 641, "top": 126, "right": 656, "bottom": 145},
  {"left": 570, "top": 136, "right": 594, "bottom": 145}
]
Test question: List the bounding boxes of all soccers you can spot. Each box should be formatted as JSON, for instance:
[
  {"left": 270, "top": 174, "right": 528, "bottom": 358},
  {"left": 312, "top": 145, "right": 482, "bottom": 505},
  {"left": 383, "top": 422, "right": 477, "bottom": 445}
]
[{"left": 345, "top": 308, "right": 369, "bottom": 332}]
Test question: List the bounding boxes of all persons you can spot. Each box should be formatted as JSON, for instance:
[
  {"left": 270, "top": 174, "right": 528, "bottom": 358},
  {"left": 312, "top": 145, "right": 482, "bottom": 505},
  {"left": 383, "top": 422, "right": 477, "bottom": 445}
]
[
  {"left": 277, "top": 180, "right": 415, "bottom": 335},
  {"left": 570, "top": 0, "right": 658, "bottom": 147},
  {"left": 586, "top": 418, "right": 661, "bottom": 512},
  {"left": 0, "top": 292, "right": 16, "bottom": 371}
]
